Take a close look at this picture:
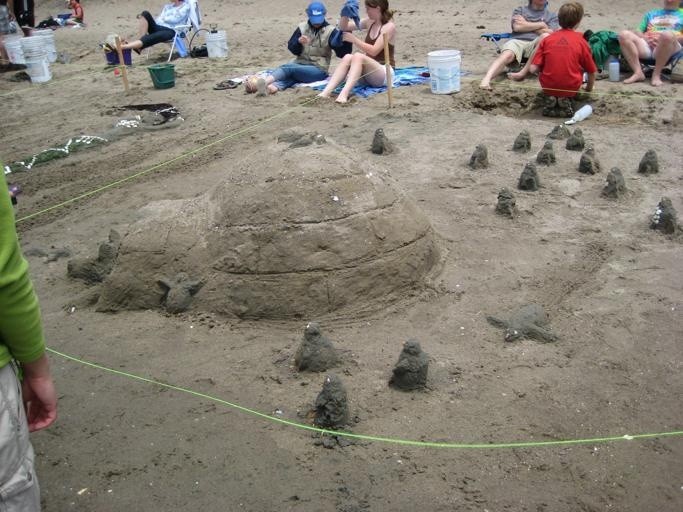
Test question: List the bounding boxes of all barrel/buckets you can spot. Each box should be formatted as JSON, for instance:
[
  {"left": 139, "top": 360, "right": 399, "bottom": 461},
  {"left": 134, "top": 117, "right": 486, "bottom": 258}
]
[
  {"left": 204, "top": 30, "right": 228, "bottom": 57},
  {"left": 4, "top": 29, "right": 58, "bottom": 84},
  {"left": 426, "top": 49, "right": 462, "bottom": 95},
  {"left": 147, "top": 64, "right": 178, "bottom": 89}
]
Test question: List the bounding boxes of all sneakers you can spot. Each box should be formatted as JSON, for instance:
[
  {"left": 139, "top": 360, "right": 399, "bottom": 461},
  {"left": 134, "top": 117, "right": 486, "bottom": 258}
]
[{"left": 543, "top": 96, "right": 574, "bottom": 117}]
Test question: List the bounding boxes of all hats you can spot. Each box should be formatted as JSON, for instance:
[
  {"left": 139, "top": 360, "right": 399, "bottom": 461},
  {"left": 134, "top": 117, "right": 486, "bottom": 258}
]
[{"left": 307, "top": 3, "right": 326, "bottom": 25}]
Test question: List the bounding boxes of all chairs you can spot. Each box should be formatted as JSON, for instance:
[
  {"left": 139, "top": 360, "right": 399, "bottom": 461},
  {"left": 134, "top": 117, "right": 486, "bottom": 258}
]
[
  {"left": 147, "top": 0, "right": 198, "bottom": 63},
  {"left": 481, "top": 33, "right": 539, "bottom": 77}
]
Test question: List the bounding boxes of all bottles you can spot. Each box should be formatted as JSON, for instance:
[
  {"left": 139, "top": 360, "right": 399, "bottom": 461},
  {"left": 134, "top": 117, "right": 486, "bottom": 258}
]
[
  {"left": 564, "top": 104, "right": 593, "bottom": 126},
  {"left": 608, "top": 59, "right": 619, "bottom": 82}
]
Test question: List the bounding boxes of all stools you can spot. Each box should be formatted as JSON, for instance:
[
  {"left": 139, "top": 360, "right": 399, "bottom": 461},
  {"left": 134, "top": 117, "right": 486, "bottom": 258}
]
[{"left": 639, "top": 50, "right": 683, "bottom": 81}]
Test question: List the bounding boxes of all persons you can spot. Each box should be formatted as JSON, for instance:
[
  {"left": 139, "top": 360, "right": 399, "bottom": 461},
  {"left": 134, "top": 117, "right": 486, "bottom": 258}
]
[
  {"left": 529, "top": 2, "right": 597, "bottom": 101},
  {"left": 479, "top": 0, "right": 560, "bottom": 92},
  {"left": 0, "top": 154, "right": 59, "bottom": 512},
  {"left": 0, "top": 0, "right": 83, "bottom": 35},
  {"left": 617, "top": 0, "right": 683, "bottom": 87},
  {"left": 99, "top": 0, "right": 190, "bottom": 55},
  {"left": 245, "top": 1, "right": 399, "bottom": 103}
]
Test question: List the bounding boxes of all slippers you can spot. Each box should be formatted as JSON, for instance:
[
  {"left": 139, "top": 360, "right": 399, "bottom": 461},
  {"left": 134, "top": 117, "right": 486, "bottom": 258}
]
[{"left": 214, "top": 80, "right": 242, "bottom": 90}]
